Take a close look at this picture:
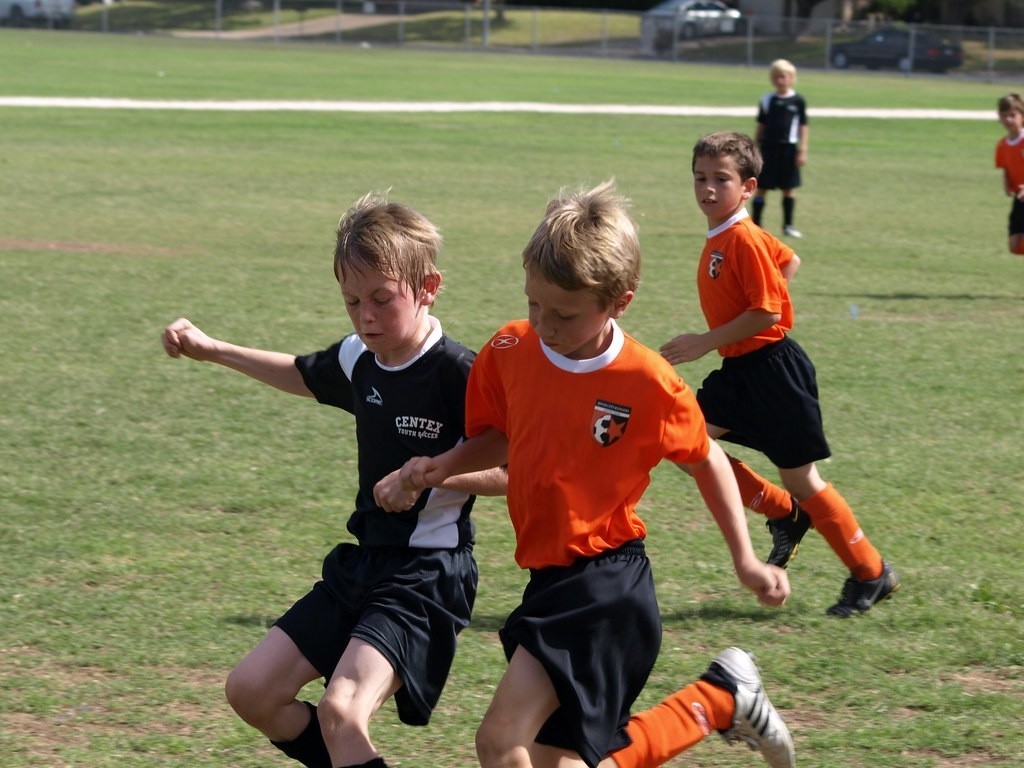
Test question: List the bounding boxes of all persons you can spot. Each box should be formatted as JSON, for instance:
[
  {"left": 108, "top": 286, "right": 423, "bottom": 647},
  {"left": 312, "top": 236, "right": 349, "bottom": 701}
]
[
  {"left": 401, "top": 177, "right": 798, "bottom": 768},
  {"left": 163, "top": 194, "right": 509, "bottom": 768},
  {"left": 992, "top": 94, "right": 1024, "bottom": 254},
  {"left": 657, "top": 131, "right": 902, "bottom": 618},
  {"left": 749, "top": 59, "right": 809, "bottom": 238}
]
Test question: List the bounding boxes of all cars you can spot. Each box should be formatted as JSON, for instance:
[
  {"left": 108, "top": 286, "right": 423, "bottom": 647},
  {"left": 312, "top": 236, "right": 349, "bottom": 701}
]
[
  {"left": 639, "top": 0, "right": 749, "bottom": 39},
  {"left": 829, "top": 25, "right": 964, "bottom": 73},
  {"left": 0, "top": 0, "right": 75, "bottom": 26}
]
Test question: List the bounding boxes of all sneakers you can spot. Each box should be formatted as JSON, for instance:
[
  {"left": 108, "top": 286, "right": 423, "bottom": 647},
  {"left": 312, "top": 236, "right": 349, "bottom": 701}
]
[
  {"left": 700, "top": 647, "right": 795, "bottom": 768},
  {"left": 826, "top": 558, "right": 901, "bottom": 618},
  {"left": 766, "top": 496, "right": 815, "bottom": 569}
]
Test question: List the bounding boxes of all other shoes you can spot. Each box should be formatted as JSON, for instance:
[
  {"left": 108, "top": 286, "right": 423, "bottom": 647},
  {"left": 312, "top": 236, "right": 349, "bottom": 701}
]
[{"left": 784, "top": 228, "right": 802, "bottom": 238}]
